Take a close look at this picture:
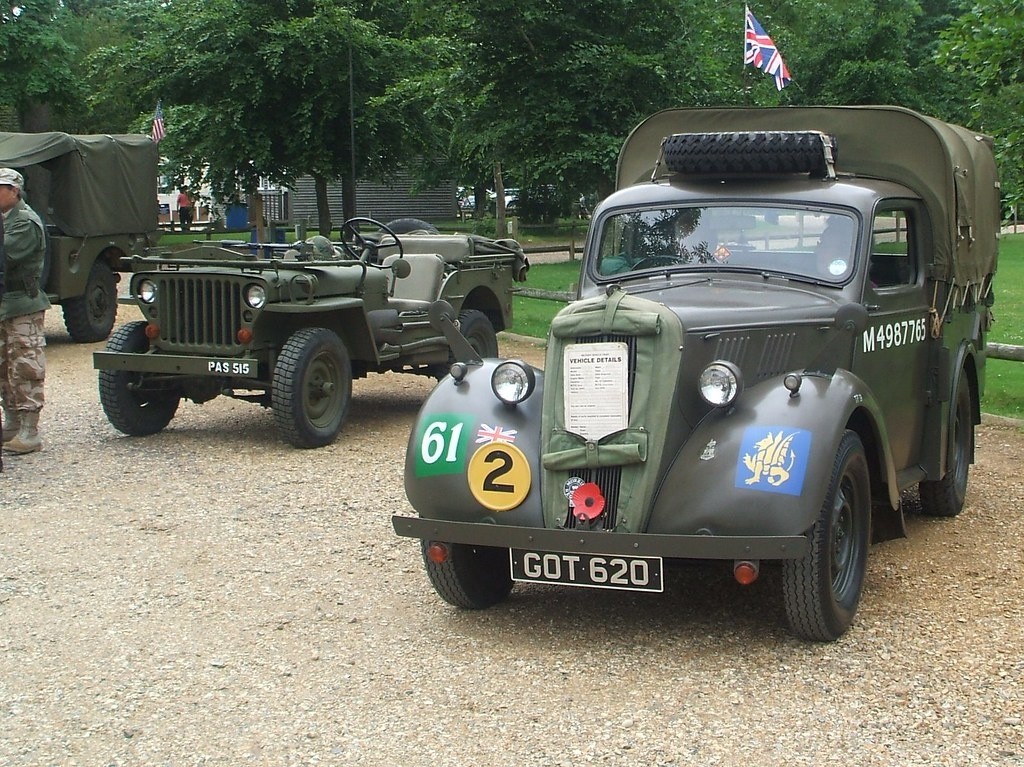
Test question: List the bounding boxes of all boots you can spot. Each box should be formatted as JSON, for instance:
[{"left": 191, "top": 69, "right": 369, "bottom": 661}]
[
  {"left": 0, "top": 409, "right": 20, "bottom": 442},
  {"left": 3, "top": 412, "right": 41, "bottom": 453}
]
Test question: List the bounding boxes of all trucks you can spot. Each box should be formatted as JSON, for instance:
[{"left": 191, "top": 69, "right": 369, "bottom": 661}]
[
  {"left": 388, "top": 101, "right": 1003, "bottom": 643},
  {"left": 0, "top": 130, "right": 161, "bottom": 348}
]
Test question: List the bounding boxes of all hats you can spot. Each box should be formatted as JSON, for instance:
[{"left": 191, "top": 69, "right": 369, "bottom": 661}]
[{"left": 0, "top": 168, "right": 24, "bottom": 190}]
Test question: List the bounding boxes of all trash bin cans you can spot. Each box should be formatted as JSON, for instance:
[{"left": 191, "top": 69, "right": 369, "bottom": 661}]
[
  {"left": 224, "top": 200, "right": 248, "bottom": 231},
  {"left": 251, "top": 228, "right": 285, "bottom": 258}
]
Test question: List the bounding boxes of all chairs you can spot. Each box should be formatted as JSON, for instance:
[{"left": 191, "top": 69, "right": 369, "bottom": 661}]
[
  {"left": 285, "top": 249, "right": 341, "bottom": 259},
  {"left": 382, "top": 253, "right": 443, "bottom": 314}
]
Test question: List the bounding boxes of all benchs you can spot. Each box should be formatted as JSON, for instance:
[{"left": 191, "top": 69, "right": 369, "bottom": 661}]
[{"left": 378, "top": 235, "right": 475, "bottom": 269}]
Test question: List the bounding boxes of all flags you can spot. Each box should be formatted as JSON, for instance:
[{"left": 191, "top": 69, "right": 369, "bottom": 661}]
[
  {"left": 152, "top": 100, "right": 166, "bottom": 143},
  {"left": 746, "top": 7, "right": 794, "bottom": 91}
]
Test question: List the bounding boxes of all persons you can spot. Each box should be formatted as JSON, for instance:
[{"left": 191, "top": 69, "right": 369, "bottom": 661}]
[
  {"left": 177, "top": 186, "right": 195, "bottom": 232},
  {"left": 600, "top": 208, "right": 718, "bottom": 277},
  {"left": 0, "top": 168, "right": 51, "bottom": 452},
  {"left": 813, "top": 216, "right": 877, "bottom": 287}
]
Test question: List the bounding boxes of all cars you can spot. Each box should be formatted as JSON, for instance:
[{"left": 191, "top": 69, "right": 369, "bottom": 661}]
[{"left": 92, "top": 213, "right": 530, "bottom": 451}]
